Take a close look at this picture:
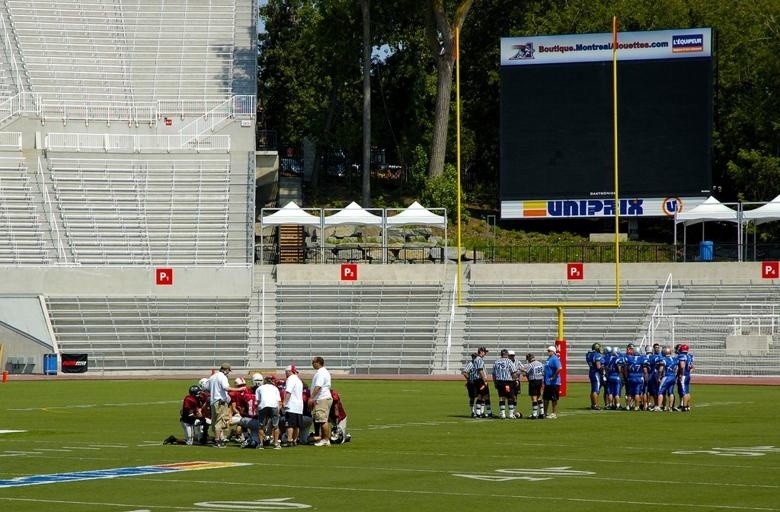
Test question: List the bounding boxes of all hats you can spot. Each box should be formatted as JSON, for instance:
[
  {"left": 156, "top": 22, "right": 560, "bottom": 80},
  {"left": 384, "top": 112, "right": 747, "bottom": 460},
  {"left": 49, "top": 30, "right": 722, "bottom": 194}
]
[
  {"left": 501, "top": 349, "right": 516, "bottom": 357},
  {"left": 284, "top": 364, "right": 300, "bottom": 374},
  {"left": 222, "top": 362, "right": 232, "bottom": 371},
  {"left": 478, "top": 346, "right": 489, "bottom": 353},
  {"left": 546, "top": 345, "right": 557, "bottom": 355},
  {"left": 470, "top": 352, "right": 477, "bottom": 358}
]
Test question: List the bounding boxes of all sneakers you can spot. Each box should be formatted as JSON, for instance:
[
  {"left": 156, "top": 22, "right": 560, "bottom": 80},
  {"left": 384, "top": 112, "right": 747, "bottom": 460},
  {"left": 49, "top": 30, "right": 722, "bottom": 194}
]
[
  {"left": 163, "top": 432, "right": 353, "bottom": 448},
  {"left": 590, "top": 403, "right": 691, "bottom": 412},
  {"left": 471, "top": 412, "right": 558, "bottom": 420}
]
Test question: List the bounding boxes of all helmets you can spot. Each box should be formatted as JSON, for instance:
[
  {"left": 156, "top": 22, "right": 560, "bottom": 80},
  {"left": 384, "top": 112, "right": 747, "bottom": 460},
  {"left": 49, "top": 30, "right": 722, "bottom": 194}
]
[
  {"left": 188, "top": 385, "right": 202, "bottom": 397},
  {"left": 234, "top": 377, "right": 247, "bottom": 389},
  {"left": 591, "top": 341, "right": 689, "bottom": 357},
  {"left": 251, "top": 373, "right": 264, "bottom": 386},
  {"left": 525, "top": 352, "right": 535, "bottom": 363},
  {"left": 198, "top": 378, "right": 210, "bottom": 389}
]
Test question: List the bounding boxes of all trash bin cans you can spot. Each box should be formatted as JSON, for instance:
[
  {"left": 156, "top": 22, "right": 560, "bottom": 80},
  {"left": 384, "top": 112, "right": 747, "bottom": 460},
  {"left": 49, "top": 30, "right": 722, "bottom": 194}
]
[
  {"left": 44, "top": 354, "right": 58, "bottom": 375},
  {"left": 700, "top": 241, "right": 713, "bottom": 261}
]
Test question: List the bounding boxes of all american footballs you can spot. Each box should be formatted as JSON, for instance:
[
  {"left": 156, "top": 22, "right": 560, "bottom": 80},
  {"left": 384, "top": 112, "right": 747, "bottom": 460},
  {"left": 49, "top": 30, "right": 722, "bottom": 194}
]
[{"left": 514, "top": 412, "right": 522, "bottom": 418}]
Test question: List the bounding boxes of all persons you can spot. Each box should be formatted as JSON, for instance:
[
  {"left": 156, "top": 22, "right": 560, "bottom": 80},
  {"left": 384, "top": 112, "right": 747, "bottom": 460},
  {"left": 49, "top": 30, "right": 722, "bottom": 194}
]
[
  {"left": 463, "top": 345, "right": 562, "bottom": 420},
  {"left": 164, "top": 356, "right": 351, "bottom": 451},
  {"left": 586, "top": 342, "right": 695, "bottom": 413}
]
